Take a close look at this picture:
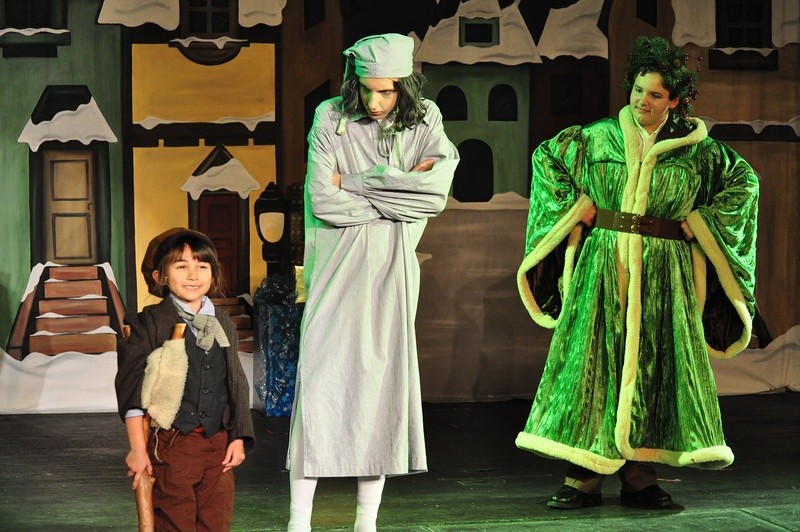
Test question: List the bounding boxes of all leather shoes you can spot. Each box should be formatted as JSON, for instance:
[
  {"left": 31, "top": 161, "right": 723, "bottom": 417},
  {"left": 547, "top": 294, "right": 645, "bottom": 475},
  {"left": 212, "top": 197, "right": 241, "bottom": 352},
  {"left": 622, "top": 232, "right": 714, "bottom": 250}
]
[
  {"left": 620, "top": 485, "right": 673, "bottom": 508},
  {"left": 547, "top": 485, "right": 603, "bottom": 508}
]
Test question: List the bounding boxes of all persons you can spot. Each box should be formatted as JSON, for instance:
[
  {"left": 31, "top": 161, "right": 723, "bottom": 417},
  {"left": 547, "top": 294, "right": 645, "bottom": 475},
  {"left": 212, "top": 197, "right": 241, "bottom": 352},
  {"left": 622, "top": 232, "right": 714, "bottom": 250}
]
[
  {"left": 116, "top": 228, "right": 255, "bottom": 531},
  {"left": 285, "top": 34, "right": 461, "bottom": 531},
  {"left": 516, "top": 34, "right": 759, "bottom": 511}
]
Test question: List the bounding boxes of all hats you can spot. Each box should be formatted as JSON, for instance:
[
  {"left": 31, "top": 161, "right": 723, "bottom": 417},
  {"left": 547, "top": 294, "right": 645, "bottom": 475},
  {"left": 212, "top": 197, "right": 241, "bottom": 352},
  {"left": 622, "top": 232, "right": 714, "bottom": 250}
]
[
  {"left": 337, "top": 33, "right": 415, "bottom": 135},
  {"left": 141, "top": 227, "right": 187, "bottom": 297}
]
